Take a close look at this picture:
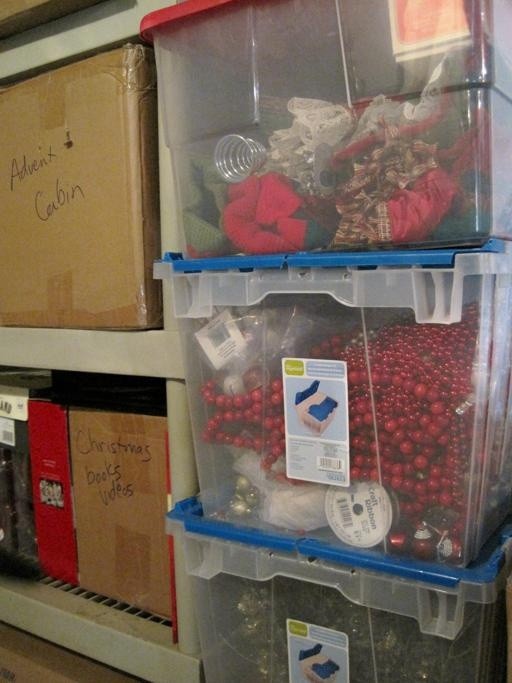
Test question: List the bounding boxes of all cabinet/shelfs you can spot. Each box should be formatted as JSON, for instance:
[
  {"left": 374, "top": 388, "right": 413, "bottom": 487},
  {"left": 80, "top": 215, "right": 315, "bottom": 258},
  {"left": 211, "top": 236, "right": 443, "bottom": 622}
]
[{"left": 2, "top": 0, "right": 204, "bottom": 683}]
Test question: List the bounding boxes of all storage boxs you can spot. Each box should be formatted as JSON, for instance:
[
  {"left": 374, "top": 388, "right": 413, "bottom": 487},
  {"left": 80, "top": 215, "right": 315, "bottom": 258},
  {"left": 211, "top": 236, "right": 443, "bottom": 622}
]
[
  {"left": 165, "top": 490, "right": 512, "bottom": 680},
  {"left": 143, "top": 249, "right": 512, "bottom": 567},
  {"left": 136, "top": 1, "right": 511, "bottom": 253},
  {"left": 4, "top": 39, "right": 166, "bottom": 337}
]
